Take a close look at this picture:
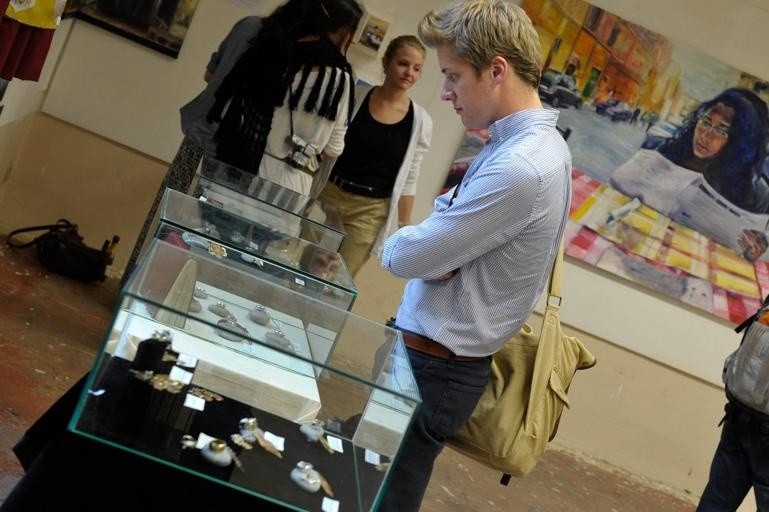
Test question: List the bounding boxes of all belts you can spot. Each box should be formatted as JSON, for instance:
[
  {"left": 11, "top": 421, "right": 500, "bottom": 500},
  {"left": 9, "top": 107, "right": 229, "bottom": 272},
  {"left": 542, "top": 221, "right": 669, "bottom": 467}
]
[
  {"left": 329, "top": 173, "right": 390, "bottom": 199},
  {"left": 401, "top": 331, "right": 492, "bottom": 365}
]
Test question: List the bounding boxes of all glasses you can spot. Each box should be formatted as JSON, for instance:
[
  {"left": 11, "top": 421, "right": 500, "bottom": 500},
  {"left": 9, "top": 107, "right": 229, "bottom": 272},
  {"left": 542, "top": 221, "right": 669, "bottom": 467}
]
[{"left": 698, "top": 117, "right": 732, "bottom": 139}]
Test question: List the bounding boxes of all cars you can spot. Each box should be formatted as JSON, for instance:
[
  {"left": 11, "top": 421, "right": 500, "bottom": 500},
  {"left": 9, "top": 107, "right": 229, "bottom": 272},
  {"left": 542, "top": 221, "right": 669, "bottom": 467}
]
[
  {"left": 539, "top": 68, "right": 583, "bottom": 109},
  {"left": 646, "top": 122, "right": 679, "bottom": 140},
  {"left": 367, "top": 32, "right": 382, "bottom": 46},
  {"left": 595, "top": 98, "right": 633, "bottom": 123}
]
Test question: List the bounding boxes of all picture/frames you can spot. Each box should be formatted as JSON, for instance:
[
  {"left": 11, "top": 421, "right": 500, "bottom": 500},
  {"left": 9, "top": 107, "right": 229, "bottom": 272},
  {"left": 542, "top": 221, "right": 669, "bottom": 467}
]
[{"left": 79, "top": 0, "right": 202, "bottom": 58}]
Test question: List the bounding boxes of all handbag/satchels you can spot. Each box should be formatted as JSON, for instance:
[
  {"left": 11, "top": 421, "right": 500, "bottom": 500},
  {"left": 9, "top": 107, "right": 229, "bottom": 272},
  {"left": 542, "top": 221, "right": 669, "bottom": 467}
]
[
  {"left": 444, "top": 320, "right": 598, "bottom": 488},
  {"left": 6, "top": 216, "right": 120, "bottom": 284}
]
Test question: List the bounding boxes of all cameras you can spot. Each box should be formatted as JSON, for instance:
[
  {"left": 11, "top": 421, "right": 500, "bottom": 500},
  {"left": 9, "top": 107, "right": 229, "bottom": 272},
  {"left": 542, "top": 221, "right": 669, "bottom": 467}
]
[{"left": 286, "top": 134, "right": 316, "bottom": 169}]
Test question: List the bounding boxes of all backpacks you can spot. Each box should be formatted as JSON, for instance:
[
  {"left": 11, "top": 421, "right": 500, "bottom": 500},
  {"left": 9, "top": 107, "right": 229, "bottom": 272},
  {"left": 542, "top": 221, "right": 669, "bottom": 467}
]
[{"left": 720, "top": 294, "right": 769, "bottom": 421}]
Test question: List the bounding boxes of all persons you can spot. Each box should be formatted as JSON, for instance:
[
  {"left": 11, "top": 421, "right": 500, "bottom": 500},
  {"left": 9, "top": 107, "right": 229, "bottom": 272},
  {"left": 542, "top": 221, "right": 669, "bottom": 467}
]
[
  {"left": 689, "top": 290, "right": 768, "bottom": 512},
  {"left": 207, "top": 0, "right": 365, "bottom": 251},
  {"left": 310, "top": 0, "right": 574, "bottom": 512},
  {"left": 119, "top": 13, "right": 268, "bottom": 292},
  {"left": 662, "top": 82, "right": 769, "bottom": 263},
  {"left": 311, "top": 32, "right": 436, "bottom": 279},
  {"left": 299, "top": 246, "right": 342, "bottom": 280}
]
